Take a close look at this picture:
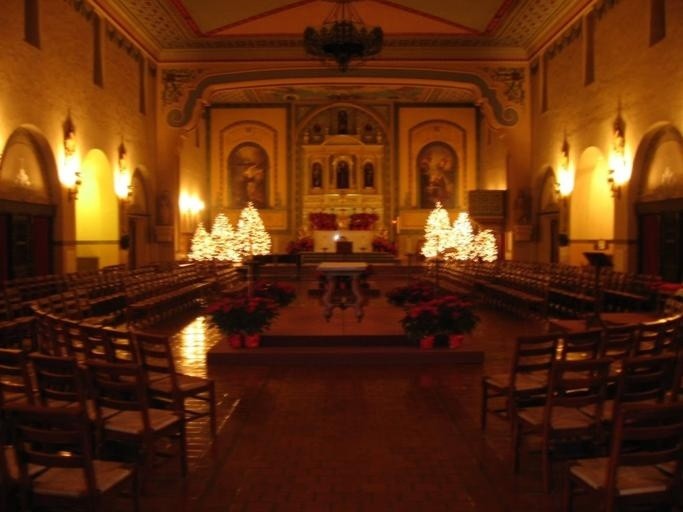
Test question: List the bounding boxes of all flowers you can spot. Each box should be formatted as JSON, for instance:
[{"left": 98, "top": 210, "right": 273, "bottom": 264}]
[
  {"left": 385, "top": 283, "right": 484, "bottom": 334},
  {"left": 205, "top": 280, "right": 296, "bottom": 336}
]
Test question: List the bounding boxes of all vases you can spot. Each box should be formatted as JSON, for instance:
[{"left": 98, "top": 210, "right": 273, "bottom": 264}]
[
  {"left": 227, "top": 331, "right": 260, "bottom": 349},
  {"left": 418, "top": 334, "right": 465, "bottom": 349}
]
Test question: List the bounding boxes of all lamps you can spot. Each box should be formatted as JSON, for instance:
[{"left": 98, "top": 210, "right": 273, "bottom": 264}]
[{"left": 301, "top": 0, "right": 385, "bottom": 71}]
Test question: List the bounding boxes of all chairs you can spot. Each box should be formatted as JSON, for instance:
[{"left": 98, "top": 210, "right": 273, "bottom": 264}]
[{"left": 416, "top": 255, "right": 679, "bottom": 512}]
